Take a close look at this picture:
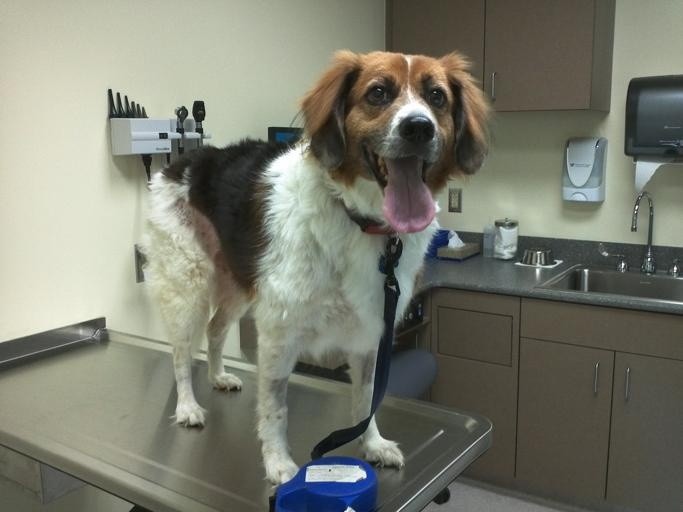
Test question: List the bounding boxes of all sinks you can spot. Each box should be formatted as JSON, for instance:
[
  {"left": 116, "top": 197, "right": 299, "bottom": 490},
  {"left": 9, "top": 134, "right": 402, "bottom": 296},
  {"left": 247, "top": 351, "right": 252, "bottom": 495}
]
[{"left": 543, "top": 264, "right": 683, "bottom": 304}]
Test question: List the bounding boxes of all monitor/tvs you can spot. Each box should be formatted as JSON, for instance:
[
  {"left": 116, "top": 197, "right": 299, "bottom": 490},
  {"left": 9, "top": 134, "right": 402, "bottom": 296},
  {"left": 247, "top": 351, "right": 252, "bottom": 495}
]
[{"left": 268, "top": 126, "right": 304, "bottom": 145}]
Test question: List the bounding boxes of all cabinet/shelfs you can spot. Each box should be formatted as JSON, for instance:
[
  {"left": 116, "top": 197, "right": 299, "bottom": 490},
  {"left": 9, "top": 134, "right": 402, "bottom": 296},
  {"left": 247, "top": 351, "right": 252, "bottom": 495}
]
[
  {"left": 512, "top": 296, "right": 683, "bottom": 512},
  {"left": 384, "top": 0, "right": 617, "bottom": 117}
]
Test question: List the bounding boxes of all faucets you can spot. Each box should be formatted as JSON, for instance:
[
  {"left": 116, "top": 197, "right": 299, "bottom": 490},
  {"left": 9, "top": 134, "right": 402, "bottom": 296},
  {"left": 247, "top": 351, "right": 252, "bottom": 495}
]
[{"left": 631, "top": 190, "right": 655, "bottom": 273}]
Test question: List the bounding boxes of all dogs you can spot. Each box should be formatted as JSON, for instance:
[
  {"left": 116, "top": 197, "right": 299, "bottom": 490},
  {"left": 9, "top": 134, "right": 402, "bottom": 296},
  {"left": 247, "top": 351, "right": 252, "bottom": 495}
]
[{"left": 136, "top": 48, "right": 501, "bottom": 488}]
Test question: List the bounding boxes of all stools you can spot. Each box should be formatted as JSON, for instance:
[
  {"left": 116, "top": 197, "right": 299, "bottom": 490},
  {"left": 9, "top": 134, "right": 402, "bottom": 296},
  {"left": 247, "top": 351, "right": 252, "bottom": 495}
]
[{"left": 383, "top": 344, "right": 450, "bottom": 505}]
